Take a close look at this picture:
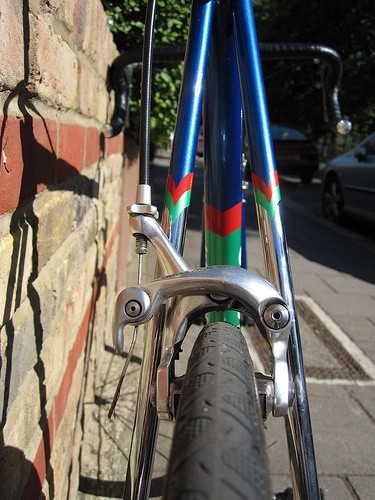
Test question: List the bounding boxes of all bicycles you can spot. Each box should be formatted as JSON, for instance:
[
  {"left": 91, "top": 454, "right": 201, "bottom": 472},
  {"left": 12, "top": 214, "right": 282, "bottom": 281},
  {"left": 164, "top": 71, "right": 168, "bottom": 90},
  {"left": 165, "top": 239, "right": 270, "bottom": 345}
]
[{"left": 107, "top": 0, "right": 353, "bottom": 500}]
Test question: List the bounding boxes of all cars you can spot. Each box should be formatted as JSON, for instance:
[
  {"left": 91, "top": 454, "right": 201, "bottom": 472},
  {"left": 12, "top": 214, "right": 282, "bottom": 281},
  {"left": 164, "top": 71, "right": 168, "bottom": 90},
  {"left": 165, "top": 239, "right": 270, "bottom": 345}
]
[
  {"left": 243, "top": 123, "right": 318, "bottom": 184},
  {"left": 324, "top": 133, "right": 375, "bottom": 231}
]
